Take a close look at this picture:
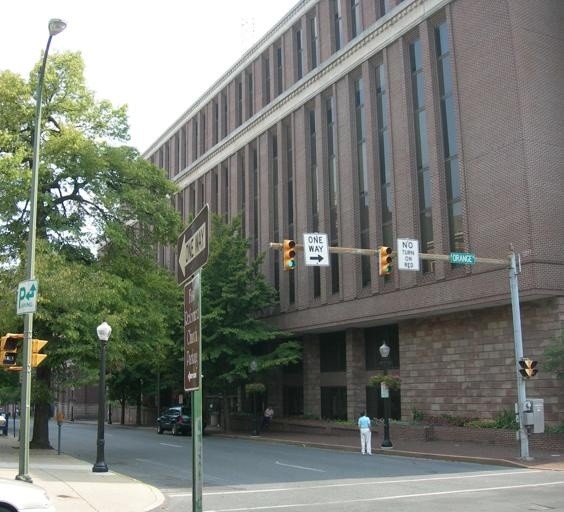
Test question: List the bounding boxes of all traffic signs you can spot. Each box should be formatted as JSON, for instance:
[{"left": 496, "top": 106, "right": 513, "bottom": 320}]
[
  {"left": 303, "top": 233, "right": 329, "bottom": 266},
  {"left": 397, "top": 237, "right": 420, "bottom": 272},
  {"left": 176, "top": 203, "right": 209, "bottom": 392}
]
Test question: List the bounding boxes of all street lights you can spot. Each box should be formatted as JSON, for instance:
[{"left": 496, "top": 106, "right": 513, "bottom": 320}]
[
  {"left": 18, "top": 19, "right": 68, "bottom": 483},
  {"left": 93, "top": 322, "right": 112, "bottom": 472},
  {"left": 71, "top": 386, "right": 75, "bottom": 421},
  {"left": 250, "top": 358, "right": 259, "bottom": 436},
  {"left": 378, "top": 342, "right": 393, "bottom": 447}
]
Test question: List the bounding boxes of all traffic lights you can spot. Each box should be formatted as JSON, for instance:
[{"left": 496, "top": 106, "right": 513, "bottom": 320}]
[
  {"left": 0, "top": 333, "right": 48, "bottom": 371},
  {"left": 283, "top": 239, "right": 297, "bottom": 270},
  {"left": 378, "top": 246, "right": 392, "bottom": 277},
  {"left": 519, "top": 358, "right": 538, "bottom": 379}
]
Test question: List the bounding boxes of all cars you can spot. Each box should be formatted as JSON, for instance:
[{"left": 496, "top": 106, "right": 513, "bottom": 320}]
[{"left": 155, "top": 406, "right": 205, "bottom": 436}]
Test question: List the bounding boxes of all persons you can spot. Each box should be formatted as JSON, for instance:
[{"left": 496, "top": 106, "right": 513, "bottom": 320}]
[
  {"left": 358, "top": 411, "right": 373, "bottom": 456},
  {"left": 264, "top": 405, "right": 274, "bottom": 428}
]
